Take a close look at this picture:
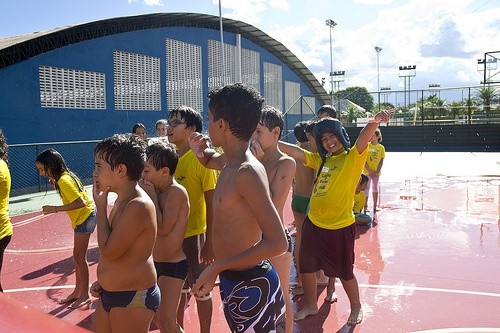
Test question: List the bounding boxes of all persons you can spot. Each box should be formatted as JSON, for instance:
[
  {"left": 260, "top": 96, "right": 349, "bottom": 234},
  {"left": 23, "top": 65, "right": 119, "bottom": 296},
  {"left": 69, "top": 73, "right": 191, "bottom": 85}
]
[
  {"left": 0, "top": 127, "right": 13, "bottom": 293},
  {"left": 167, "top": 105, "right": 220, "bottom": 333},
  {"left": 364, "top": 129, "right": 385, "bottom": 212},
  {"left": 188, "top": 82, "right": 289, "bottom": 333},
  {"left": 292, "top": 104, "right": 338, "bottom": 303},
  {"left": 249, "top": 105, "right": 296, "bottom": 333},
  {"left": 353, "top": 174, "right": 372, "bottom": 226},
  {"left": 147, "top": 119, "right": 169, "bottom": 146},
  {"left": 90, "top": 132, "right": 161, "bottom": 333},
  {"left": 277, "top": 109, "right": 396, "bottom": 325},
  {"left": 36, "top": 148, "right": 97, "bottom": 308},
  {"left": 138, "top": 143, "right": 190, "bottom": 333}
]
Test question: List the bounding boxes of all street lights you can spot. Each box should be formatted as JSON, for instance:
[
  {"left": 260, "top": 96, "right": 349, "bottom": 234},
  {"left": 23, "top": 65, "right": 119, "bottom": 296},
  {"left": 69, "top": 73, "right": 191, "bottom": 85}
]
[
  {"left": 374, "top": 46, "right": 383, "bottom": 126},
  {"left": 325, "top": 18, "right": 338, "bottom": 107}
]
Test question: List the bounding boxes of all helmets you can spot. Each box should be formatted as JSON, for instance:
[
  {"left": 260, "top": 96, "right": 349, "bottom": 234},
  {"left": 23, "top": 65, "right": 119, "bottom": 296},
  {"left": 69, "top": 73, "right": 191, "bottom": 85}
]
[{"left": 313, "top": 118, "right": 350, "bottom": 160}]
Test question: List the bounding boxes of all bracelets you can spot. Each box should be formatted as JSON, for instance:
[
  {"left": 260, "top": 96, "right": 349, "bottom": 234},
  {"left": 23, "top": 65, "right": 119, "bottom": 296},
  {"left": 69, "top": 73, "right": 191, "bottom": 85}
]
[{"left": 55, "top": 206, "right": 57, "bottom": 213}]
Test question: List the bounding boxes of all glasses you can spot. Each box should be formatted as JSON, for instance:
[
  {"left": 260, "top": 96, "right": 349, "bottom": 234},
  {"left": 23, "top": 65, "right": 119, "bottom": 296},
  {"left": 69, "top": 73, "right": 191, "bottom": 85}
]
[{"left": 165, "top": 120, "right": 186, "bottom": 126}]
